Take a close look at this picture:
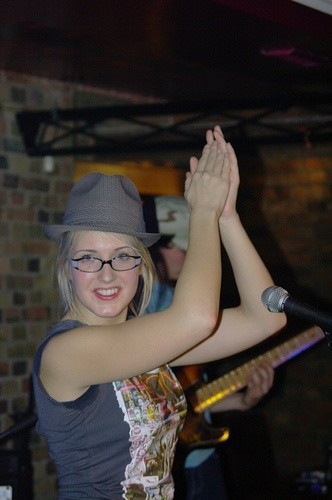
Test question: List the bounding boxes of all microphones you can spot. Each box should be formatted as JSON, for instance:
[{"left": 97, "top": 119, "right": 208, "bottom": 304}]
[{"left": 261, "top": 285, "right": 332, "bottom": 332}]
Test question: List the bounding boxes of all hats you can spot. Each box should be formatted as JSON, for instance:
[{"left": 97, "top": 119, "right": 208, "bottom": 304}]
[
  {"left": 41, "top": 173, "right": 160, "bottom": 247},
  {"left": 144, "top": 193, "right": 190, "bottom": 249}
]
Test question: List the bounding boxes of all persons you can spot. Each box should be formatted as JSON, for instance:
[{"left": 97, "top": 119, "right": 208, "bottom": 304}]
[
  {"left": 124, "top": 196, "right": 276, "bottom": 500},
  {"left": 31, "top": 123, "right": 287, "bottom": 500}
]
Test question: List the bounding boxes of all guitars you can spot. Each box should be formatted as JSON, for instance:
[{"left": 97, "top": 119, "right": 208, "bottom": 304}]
[{"left": 172, "top": 326, "right": 326, "bottom": 473}]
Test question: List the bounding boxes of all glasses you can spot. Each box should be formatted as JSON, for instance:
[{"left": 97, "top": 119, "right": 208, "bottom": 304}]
[{"left": 68, "top": 256, "right": 142, "bottom": 273}]
